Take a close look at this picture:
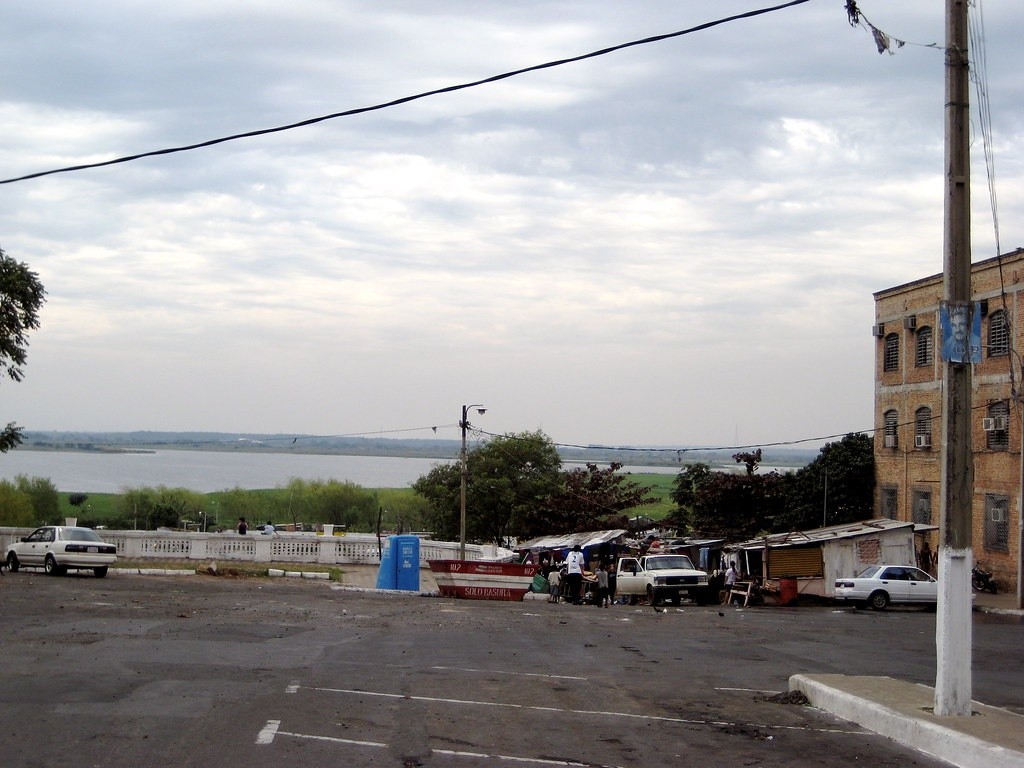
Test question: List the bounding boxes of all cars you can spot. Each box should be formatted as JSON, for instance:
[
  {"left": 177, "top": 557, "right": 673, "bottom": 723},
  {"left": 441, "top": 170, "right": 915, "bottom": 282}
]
[
  {"left": 4, "top": 525, "right": 118, "bottom": 578},
  {"left": 833, "top": 565, "right": 978, "bottom": 610}
]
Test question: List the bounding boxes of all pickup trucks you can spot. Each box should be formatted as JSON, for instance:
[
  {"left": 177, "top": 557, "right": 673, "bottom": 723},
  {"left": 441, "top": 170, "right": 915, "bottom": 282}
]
[{"left": 615, "top": 554, "right": 710, "bottom": 606}]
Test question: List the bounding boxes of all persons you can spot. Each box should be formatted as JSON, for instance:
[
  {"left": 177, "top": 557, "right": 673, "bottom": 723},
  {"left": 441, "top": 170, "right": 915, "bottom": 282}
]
[
  {"left": 543, "top": 535, "right": 662, "bottom": 608},
  {"left": 261, "top": 521, "right": 278, "bottom": 535},
  {"left": 915, "top": 543, "right": 939, "bottom": 580},
  {"left": 721, "top": 560, "right": 741, "bottom": 606},
  {"left": 237, "top": 517, "right": 249, "bottom": 535},
  {"left": 941, "top": 307, "right": 982, "bottom": 363}
]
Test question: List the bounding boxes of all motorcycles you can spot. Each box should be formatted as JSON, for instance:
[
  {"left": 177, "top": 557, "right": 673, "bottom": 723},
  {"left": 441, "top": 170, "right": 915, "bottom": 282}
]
[{"left": 972, "top": 560, "right": 999, "bottom": 595}]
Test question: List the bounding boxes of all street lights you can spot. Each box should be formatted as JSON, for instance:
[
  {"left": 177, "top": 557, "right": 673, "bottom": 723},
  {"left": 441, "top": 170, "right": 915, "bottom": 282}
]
[
  {"left": 213, "top": 500, "right": 219, "bottom": 525},
  {"left": 199, "top": 511, "right": 206, "bottom": 532},
  {"left": 460, "top": 403, "right": 487, "bottom": 561}
]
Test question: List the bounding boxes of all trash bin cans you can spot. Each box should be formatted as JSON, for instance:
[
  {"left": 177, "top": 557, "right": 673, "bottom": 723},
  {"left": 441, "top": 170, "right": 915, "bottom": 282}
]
[
  {"left": 377, "top": 535, "right": 420, "bottom": 592},
  {"left": 780, "top": 575, "right": 798, "bottom": 607}
]
